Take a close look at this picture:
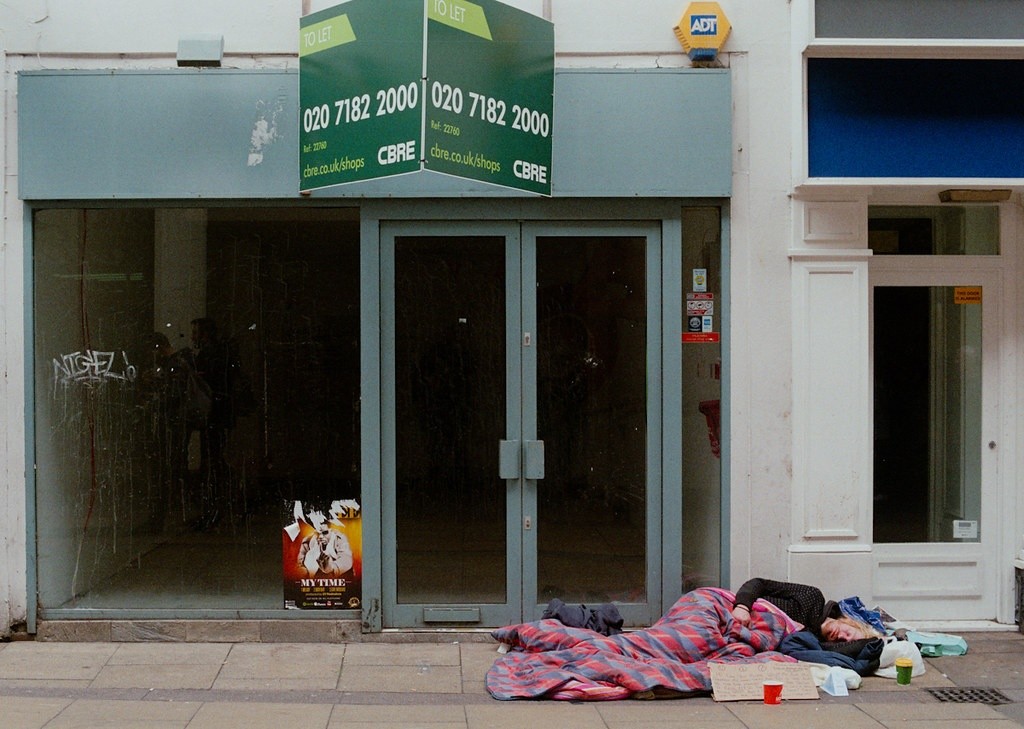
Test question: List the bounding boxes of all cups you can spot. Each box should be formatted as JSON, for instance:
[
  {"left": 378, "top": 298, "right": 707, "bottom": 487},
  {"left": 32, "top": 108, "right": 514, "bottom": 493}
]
[
  {"left": 762, "top": 680, "right": 783, "bottom": 705},
  {"left": 896, "top": 658, "right": 913, "bottom": 686}
]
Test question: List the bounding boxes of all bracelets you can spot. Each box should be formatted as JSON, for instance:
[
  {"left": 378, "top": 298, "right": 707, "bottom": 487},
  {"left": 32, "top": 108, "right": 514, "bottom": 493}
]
[{"left": 736, "top": 605, "right": 749, "bottom": 612}]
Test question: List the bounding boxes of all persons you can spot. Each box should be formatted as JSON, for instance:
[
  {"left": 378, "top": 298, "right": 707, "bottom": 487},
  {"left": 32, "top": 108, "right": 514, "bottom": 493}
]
[
  {"left": 732, "top": 578, "right": 882, "bottom": 643},
  {"left": 298, "top": 524, "right": 353, "bottom": 579},
  {"left": 132, "top": 316, "right": 230, "bottom": 537}
]
